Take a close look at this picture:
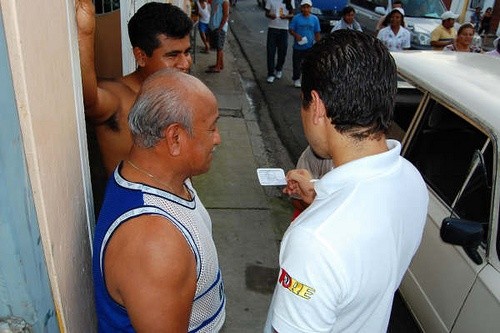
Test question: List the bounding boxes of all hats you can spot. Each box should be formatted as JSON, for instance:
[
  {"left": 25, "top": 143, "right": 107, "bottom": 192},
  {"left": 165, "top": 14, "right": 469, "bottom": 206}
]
[
  {"left": 300, "top": 0, "right": 312, "bottom": 6},
  {"left": 442, "top": 12, "right": 459, "bottom": 20},
  {"left": 393, "top": 7, "right": 404, "bottom": 16}
]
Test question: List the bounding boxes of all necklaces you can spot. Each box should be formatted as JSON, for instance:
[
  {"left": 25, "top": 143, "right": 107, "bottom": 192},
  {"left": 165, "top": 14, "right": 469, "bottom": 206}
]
[{"left": 126, "top": 158, "right": 192, "bottom": 200}]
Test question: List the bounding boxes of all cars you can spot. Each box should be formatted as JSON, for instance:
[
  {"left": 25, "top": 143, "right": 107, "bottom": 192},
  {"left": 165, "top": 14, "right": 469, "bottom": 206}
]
[
  {"left": 347, "top": 0, "right": 482, "bottom": 52},
  {"left": 387, "top": 50, "right": 500, "bottom": 333}
]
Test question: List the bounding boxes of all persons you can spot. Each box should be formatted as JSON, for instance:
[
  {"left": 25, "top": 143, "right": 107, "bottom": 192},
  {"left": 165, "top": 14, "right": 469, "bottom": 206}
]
[
  {"left": 197, "top": 0, "right": 213, "bottom": 53},
  {"left": 262, "top": 28, "right": 430, "bottom": 333},
  {"left": 204, "top": 0, "right": 229, "bottom": 74},
  {"left": 75, "top": 0, "right": 195, "bottom": 181},
  {"left": 263, "top": 0, "right": 500, "bottom": 88},
  {"left": 93, "top": 68, "right": 227, "bottom": 333}
]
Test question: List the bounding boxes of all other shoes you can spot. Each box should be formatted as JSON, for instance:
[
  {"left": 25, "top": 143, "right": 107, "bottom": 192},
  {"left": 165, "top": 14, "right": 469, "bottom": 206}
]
[
  {"left": 276, "top": 72, "right": 282, "bottom": 78},
  {"left": 205, "top": 68, "right": 220, "bottom": 72},
  {"left": 209, "top": 65, "right": 224, "bottom": 69},
  {"left": 267, "top": 76, "right": 275, "bottom": 82},
  {"left": 294, "top": 80, "right": 301, "bottom": 87}
]
